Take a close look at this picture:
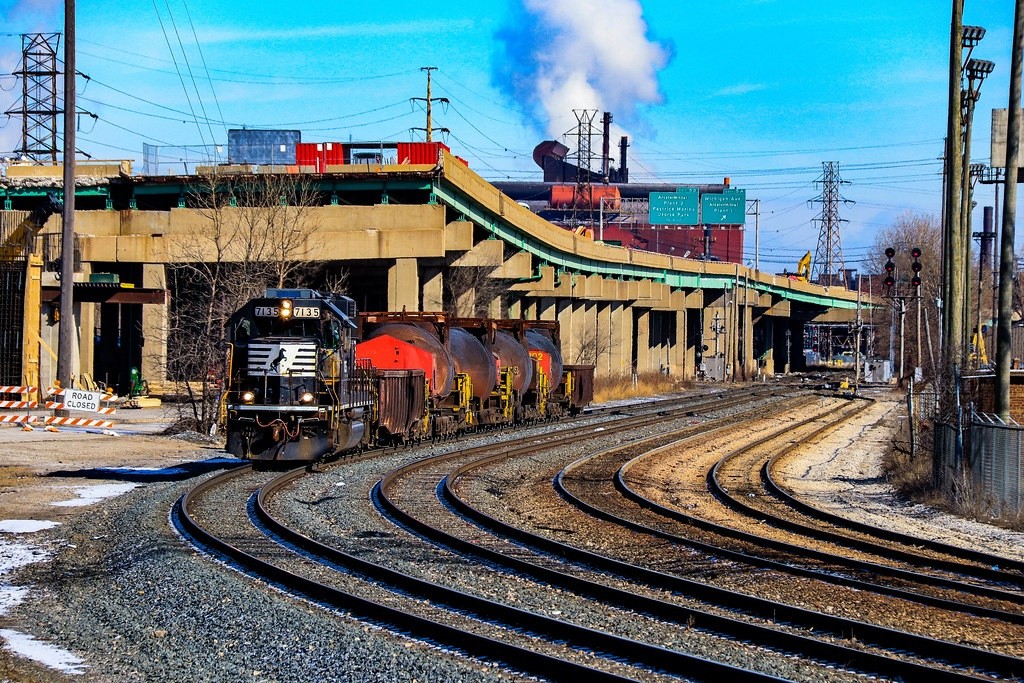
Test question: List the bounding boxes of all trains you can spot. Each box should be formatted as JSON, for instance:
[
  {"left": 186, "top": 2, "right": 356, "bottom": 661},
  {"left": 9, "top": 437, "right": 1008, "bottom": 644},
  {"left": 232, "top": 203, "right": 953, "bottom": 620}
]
[{"left": 217, "top": 290, "right": 595, "bottom": 464}]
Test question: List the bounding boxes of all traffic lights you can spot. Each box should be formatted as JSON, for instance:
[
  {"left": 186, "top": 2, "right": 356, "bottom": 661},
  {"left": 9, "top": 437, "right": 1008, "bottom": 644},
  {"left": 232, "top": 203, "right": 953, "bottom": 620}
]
[
  {"left": 912, "top": 248, "right": 922, "bottom": 286},
  {"left": 885, "top": 248, "right": 896, "bottom": 286}
]
